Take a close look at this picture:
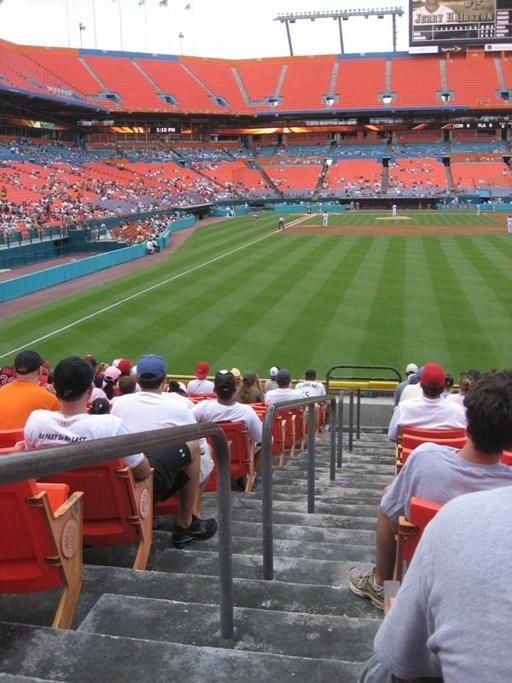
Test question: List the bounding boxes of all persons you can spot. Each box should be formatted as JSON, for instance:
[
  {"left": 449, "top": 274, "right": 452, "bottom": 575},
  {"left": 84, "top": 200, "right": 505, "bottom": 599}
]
[
  {"left": 266, "top": 371, "right": 309, "bottom": 406},
  {"left": 24, "top": 356, "right": 219, "bottom": 550},
  {"left": 192, "top": 369, "right": 263, "bottom": 491},
  {"left": 131, "top": 362, "right": 140, "bottom": 385},
  {"left": 85, "top": 354, "right": 97, "bottom": 368},
  {"left": 440, "top": 372, "right": 456, "bottom": 398},
  {"left": 185, "top": 361, "right": 215, "bottom": 394},
  {"left": 296, "top": 369, "right": 328, "bottom": 397},
  {"left": 0, "top": 351, "right": 61, "bottom": 429},
  {"left": 447, "top": 379, "right": 471, "bottom": 427},
  {"left": 229, "top": 367, "right": 244, "bottom": 400},
  {"left": 34, "top": 360, "right": 57, "bottom": 393},
  {"left": 112, "top": 358, "right": 133, "bottom": 376},
  {"left": 100, "top": 367, "right": 123, "bottom": 399},
  {"left": 94, "top": 359, "right": 108, "bottom": 386},
  {"left": 120, "top": 376, "right": 141, "bottom": 395},
  {"left": 0, "top": 367, "right": 16, "bottom": 386},
  {"left": 410, "top": 1, "right": 460, "bottom": 24},
  {"left": 109, "top": 353, "right": 216, "bottom": 506},
  {"left": 459, "top": 370, "right": 471, "bottom": 382},
  {"left": 241, "top": 372, "right": 266, "bottom": 406},
  {"left": 346, "top": 370, "right": 512, "bottom": 613},
  {"left": 392, "top": 363, "right": 419, "bottom": 406},
  {"left": 89, "top": 397, "right": 113, "bottom": 414},
  {"left": 359, "top": 483, "right": 512, "bottom": 682},
  {"left": 267, "top": 363, "right": 279, "bottom": 391},
  {"left": 191, "top": 141, "right": 512, "bottom": 233},
  {"left": 387, "top": 362, "right": 472, "bottom": 442},
  {"left": 396, "top": 366, "right": 424, "bottom": 402},
  {"left": 160, "top": 380, "right": 195, "bottom": 410},
  {"left": 1, "top": 139, "right": 189, "bottom": 254}
]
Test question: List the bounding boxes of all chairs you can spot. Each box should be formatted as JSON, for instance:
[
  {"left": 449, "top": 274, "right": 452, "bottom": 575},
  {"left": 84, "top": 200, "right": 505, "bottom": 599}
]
[{"left": 381, "top": 419, "right": 511, "bottom": 624}]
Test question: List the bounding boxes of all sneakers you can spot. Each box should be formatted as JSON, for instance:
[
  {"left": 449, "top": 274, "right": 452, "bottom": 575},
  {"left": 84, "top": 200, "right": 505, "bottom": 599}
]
[{"left": 171, "top": 515, "right": 217, "bottom": 549}]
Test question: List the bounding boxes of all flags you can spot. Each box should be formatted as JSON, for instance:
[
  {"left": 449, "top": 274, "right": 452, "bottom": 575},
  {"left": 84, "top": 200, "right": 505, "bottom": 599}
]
[{"left": 54, "top": 0, "right": 197, "bottom": 56}]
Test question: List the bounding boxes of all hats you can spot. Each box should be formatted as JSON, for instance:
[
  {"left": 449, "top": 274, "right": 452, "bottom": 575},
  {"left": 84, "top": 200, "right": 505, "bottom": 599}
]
[
  {"left": 112, "top": 360, "right": 119, "bottom": 366},
  {"left": 231, "top": 368, "right": 243, "bottom": 383},
  {"left": 269, "top": 366, "right": 279, "bottom": 379},
  {"left": 406, "top": 363, "right": 418, "bottom": 375},
  {"left": 244, "top": 371, "right": 256, "bottom": 384},
  {"left": 349, "top": 567, "right": 385, "bottom": 611},
  {"left": 305, "top": 369, "right": 316, "bottom": 376},
  {"left": 118, "top": 358, "right": 134, "bottom": 371},
  {"left": 54, "top": 357, "right": 96, "bottom": 400},
  {"left": 15, "top": 351, "right": 45, "bottom": 373},
  {"left": 276, "top": 369, "right": 291, "bottom": 385},
  {"left": 421, "top": 363, "right": 445, "bottom": 388},
  {"left": 214, "top": 370, "right": 235, "bottom": 394},
  {"left": 104, "top": 366, "right": 122, "bottom": 381},
  {"left": 137, "top": 355, "right": 167, "bottom": 382},
  {"left": 196, "top": 362, "right": 208, "bottom": 378}
]
